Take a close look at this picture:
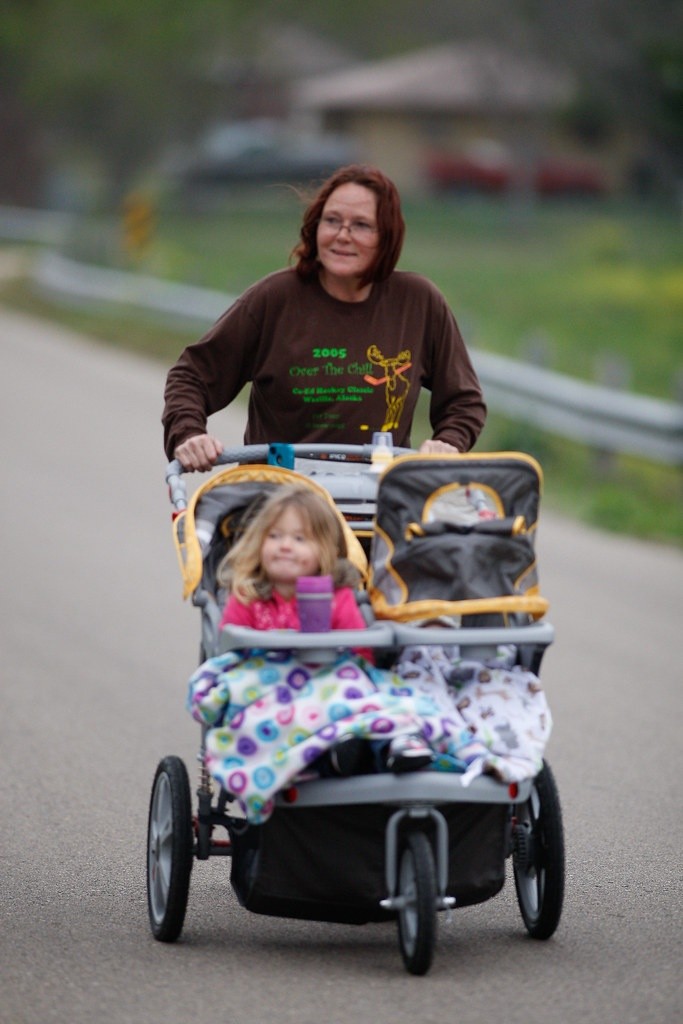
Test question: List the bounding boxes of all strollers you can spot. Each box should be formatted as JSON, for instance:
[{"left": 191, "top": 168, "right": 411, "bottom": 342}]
[{"left": 146, "top": 442, "right": 567, "bottom": 976}]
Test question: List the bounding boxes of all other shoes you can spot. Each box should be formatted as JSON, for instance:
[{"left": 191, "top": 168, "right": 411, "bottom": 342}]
[{"left": 386, "top": 736, "right": 432, "bottom": 767}]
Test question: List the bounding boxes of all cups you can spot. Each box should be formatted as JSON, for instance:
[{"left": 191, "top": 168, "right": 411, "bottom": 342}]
[{"left": 295, "top": 574, "right": 334, "bottom": 632}]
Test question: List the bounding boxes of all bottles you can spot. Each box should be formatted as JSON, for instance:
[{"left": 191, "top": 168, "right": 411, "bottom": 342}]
[{"left": 371, "top": 432, "right": 394, "bottom": 473}]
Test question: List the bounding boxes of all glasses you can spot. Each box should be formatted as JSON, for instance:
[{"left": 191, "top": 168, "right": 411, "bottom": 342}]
[{"left": 315, "top": 216, "right": 378, "bottom": 238}]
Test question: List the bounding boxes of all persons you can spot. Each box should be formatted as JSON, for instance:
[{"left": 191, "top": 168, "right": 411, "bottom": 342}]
[
  {"left": 215, "top": 485, "right": 434, "bottom": 776},
  {"left": 161, "top": 164, "right": 487, "bottom": 473}
]
